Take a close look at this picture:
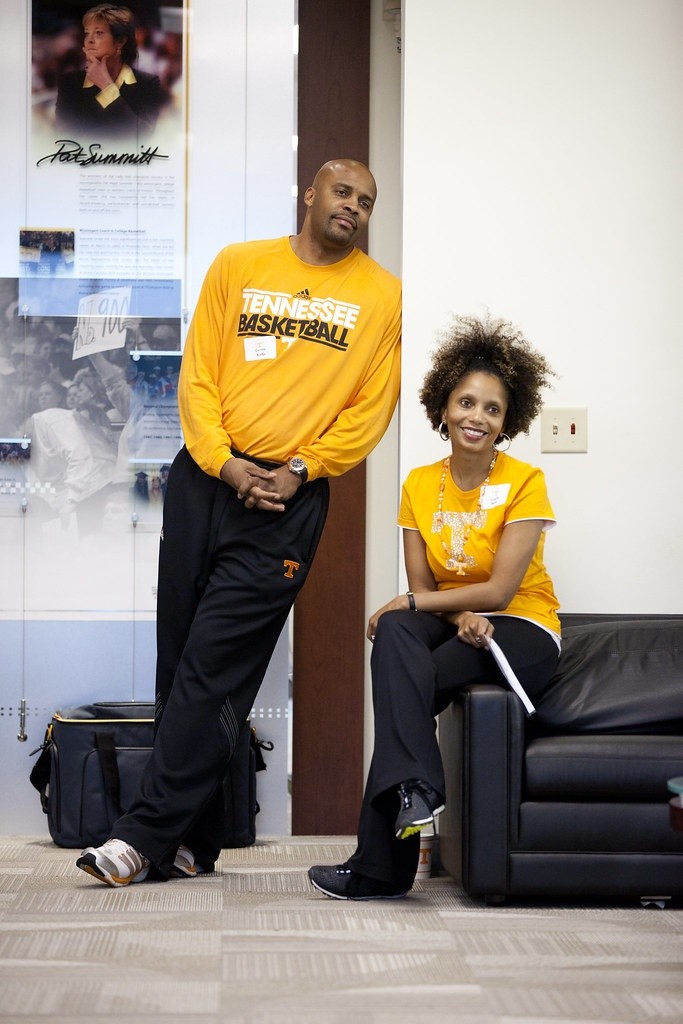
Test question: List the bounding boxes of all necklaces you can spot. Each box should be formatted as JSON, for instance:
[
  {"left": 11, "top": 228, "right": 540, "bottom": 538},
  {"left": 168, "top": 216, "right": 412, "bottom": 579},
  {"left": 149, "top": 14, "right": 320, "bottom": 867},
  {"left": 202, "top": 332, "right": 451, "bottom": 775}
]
[{"left": 436, "top": 447, "right": 499, "bottom": 564}]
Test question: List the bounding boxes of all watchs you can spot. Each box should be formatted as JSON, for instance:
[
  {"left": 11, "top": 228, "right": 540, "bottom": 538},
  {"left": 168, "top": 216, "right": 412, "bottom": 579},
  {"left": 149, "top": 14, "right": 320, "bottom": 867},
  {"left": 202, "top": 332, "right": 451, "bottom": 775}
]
[{"left": 287, "top": 457, "right": 308, "bottom": 484}]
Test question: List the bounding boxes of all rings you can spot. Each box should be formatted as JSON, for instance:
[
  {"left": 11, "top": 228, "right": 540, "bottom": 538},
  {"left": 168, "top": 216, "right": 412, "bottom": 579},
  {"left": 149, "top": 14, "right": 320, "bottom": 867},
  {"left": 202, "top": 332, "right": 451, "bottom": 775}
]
[{"left": 475, "top": 637, "right": 482, "bottom": 643}]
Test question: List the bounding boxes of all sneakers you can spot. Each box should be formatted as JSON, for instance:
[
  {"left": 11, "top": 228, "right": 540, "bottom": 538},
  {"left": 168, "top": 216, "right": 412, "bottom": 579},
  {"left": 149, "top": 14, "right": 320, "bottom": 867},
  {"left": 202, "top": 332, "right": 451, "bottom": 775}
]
[
  {"left": 308, "top": 865, "right": 407, "bottom": 901},
  {"left": 75, "top": 839, "right": 151, "bottom": 887},
  {"left": 172, "top": 845, "right": 206, "bottom": 878},
  {"left": 395, "top": 782, "right": 447, "bottom": 841}
]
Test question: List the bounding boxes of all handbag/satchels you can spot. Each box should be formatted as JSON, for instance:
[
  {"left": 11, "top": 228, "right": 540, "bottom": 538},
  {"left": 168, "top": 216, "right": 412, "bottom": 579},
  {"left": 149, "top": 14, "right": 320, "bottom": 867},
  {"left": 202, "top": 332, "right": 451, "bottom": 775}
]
[{"left": 33, "top": 699, "right": 268, "bottom": 848}]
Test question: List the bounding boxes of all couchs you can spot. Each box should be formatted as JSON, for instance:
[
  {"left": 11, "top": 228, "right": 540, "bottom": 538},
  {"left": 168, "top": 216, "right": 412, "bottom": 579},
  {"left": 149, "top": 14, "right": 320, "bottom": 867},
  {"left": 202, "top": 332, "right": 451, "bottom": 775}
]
[{"left": 434, "top": 606, "right": 683, "bottom": 912}]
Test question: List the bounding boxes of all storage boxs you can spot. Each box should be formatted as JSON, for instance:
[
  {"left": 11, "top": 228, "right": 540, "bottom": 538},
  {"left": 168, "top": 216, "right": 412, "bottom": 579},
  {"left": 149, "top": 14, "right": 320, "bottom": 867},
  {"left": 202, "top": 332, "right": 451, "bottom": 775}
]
[{"left": 27, "top": 697, "right": 274, "bottom": 851}]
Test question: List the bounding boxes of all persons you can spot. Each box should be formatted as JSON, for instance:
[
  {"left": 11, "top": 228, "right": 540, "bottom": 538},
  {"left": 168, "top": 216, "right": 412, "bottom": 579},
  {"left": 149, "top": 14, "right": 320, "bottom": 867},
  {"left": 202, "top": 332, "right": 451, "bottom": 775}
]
[
  {"left": 309, "top": 313, "right": 562, "bottom": 901},
  {"left": 75, "top": 160, "right": 402, "bottom": 889},
  {"left": 1, "top": 300, "right": 180, "bottom": 520},
  {"left": 55, "top": 3, "right": 161, "bottom": 143}
]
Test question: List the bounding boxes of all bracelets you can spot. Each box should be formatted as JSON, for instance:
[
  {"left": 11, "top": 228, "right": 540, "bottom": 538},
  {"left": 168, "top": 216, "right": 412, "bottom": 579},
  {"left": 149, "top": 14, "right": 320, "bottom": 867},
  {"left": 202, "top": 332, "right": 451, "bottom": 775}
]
[{"left": 406, "top": 591, "right": 418, "bottom": 613}]
